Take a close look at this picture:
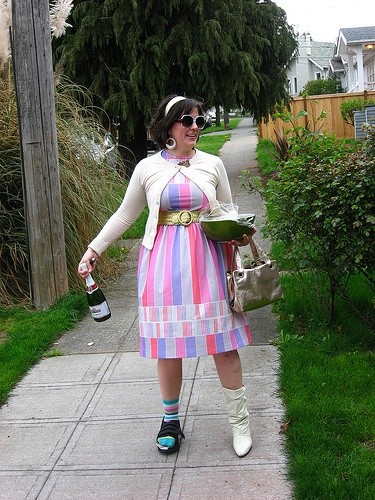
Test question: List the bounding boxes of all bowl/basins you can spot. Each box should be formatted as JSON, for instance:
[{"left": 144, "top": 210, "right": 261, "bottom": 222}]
[{"left": 199, "top": 214, "right": 256, "bottom": 244}]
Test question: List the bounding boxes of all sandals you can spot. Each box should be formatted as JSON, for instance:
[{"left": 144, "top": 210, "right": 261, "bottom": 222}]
[{"left": 156, "top": 415, "right": 185, "bottom": 453}]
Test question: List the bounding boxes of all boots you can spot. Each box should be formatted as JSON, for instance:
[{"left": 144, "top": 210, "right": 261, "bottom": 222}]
[{"left": 221, "top": 384, "right": 252, "bottom": 458}]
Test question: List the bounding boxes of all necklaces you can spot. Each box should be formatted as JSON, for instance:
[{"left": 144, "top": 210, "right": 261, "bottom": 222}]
[{"left": 167, "top": 153, "right": 190, "bottom": 167}]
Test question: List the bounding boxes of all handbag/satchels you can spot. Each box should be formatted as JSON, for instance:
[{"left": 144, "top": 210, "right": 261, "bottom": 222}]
[{"left": 227, "top": 236, "right": 284, "bottom": 313}]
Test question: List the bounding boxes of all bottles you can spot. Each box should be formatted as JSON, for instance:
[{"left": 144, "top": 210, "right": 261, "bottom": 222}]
[{"left": 79, "top": 263, "right": 111, "bottom": 322}]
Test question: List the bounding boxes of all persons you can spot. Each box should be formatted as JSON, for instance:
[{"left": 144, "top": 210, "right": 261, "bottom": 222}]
[{"left": 78, "top": 94, "right": 258, "bottom": 459}]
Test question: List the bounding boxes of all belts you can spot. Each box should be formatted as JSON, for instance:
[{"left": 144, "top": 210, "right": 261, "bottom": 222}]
[{"left": 158, "top": 210, "right": 211, "bottom": 226}]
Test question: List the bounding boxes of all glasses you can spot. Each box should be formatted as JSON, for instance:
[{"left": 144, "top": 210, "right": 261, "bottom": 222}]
[{"left": 177, "top": 115, "right": 206, "bottom": 129}]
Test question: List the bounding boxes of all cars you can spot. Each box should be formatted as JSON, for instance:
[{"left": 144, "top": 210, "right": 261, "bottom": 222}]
[
  {"left": 206, "top": 107, "right": 235, "bottom": 128},
  {"left": 74, "top": 130, "right": 120, "bottom": 176}
]
